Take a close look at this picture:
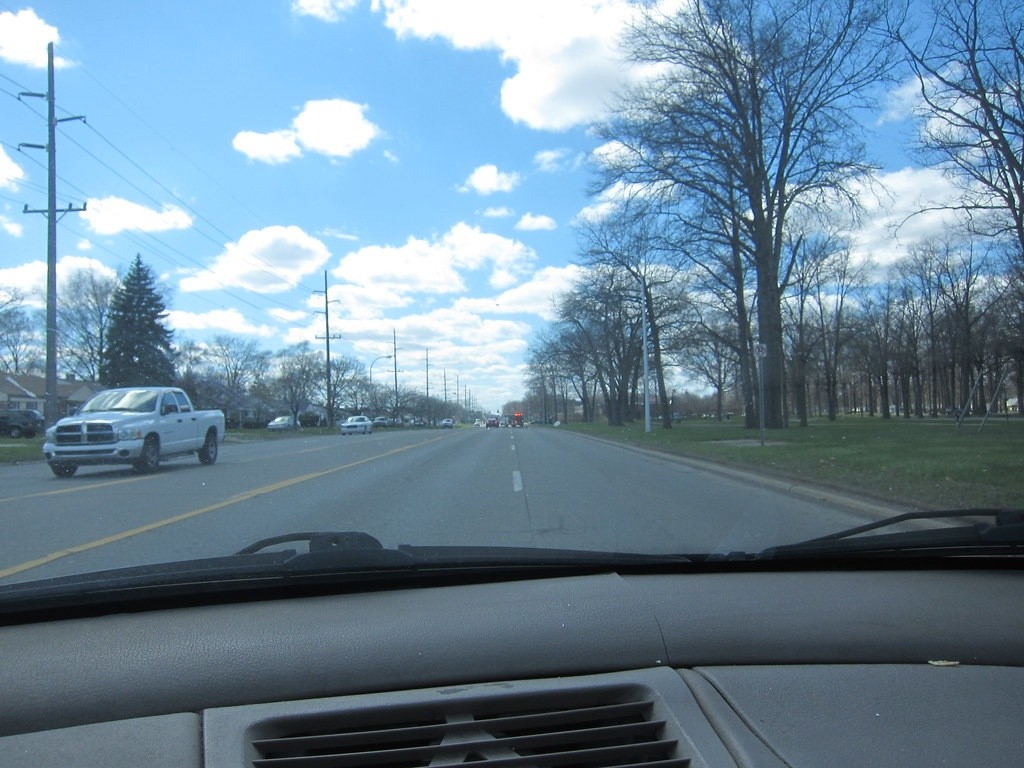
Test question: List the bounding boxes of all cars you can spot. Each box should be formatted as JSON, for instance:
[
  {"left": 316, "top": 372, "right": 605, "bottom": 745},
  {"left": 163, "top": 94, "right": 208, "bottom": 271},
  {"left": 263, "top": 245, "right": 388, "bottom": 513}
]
[
  {"left": 341, "top": 415, "right": 373, "bottom": 435},
  {"left": 372, "top": 415, "right": 437, "bottom": 428},
  {"left": 474, "top": 412, "right": 557, "bottom": 428},
  {"left": 878, "top": 399, "right": 1021, "bottom": 415},
  {"left": 267, "top": 416, "right": 301, "bottom": 432},
  {"left": 653, "top": 409, "right": 732, "bottom": 422}
]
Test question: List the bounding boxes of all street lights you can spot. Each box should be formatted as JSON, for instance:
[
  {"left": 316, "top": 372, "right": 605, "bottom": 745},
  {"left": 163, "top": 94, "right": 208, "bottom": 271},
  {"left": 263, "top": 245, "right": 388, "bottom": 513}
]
[
  {"left": 369, "top": 355, "right": 391, "bottom": 384},
  {"left": 589, "top": 248, "right": 651, "bottom": 433}
]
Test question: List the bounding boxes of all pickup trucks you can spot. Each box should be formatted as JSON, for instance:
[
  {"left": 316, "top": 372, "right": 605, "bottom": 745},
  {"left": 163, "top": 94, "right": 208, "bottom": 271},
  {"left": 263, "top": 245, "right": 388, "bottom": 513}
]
[{"left": 43, "top": 387, "right": 226, "bottom": 477}]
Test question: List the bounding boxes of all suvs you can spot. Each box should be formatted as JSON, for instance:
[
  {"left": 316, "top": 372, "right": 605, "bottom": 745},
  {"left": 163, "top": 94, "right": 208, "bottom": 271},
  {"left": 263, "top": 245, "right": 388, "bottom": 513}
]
[
  {"left": 441, "top": 419, "right": 453, "bottom": 428},
  {"left": 0, "top": 408, "right": 46, "bottom": 438}
]
